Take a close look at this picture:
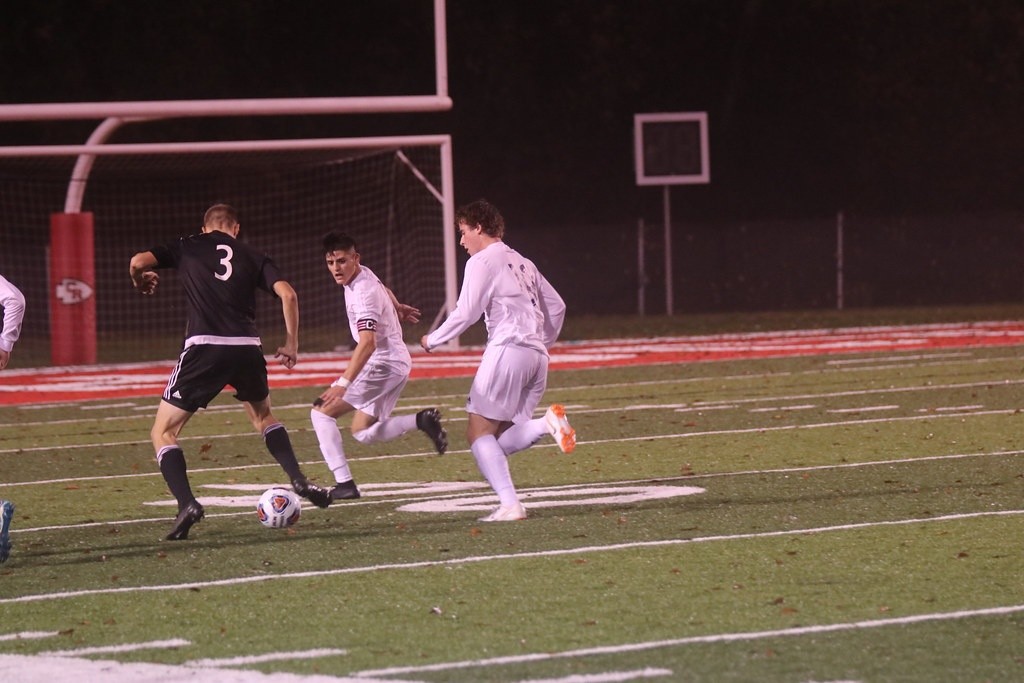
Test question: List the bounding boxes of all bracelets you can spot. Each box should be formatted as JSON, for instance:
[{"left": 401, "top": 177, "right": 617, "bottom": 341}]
[{"left": 336, "top": 376, "right": 351, "bottom": 389}]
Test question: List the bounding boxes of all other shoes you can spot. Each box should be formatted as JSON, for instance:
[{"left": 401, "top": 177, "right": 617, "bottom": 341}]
[
  {"left": 167, "top": 500, "right": 204, "bottom": 541},
  {"left": 292, "top": 477, "right": 332, "bottom": 507},
  {"left": 0, "top": 502, "right": 14, "bottom": 565}
]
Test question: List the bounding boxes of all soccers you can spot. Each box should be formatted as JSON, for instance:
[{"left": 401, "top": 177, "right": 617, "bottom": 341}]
[{"left": 256, "top": 486, "right": 302, "bottom": 530}]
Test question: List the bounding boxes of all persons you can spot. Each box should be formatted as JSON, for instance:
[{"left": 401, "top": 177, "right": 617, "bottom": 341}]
[
  {"left": 304, "top": 230, "right": 449, "bottom": 501},
  {"left": 0, "top": 275, "right": 26, "bottom": 564},
  {"left": 420, "top": 198, "right": 577, "bottom": 523},
  {"left": 129, "top": 202, "right": 332, "bottom": 539}
]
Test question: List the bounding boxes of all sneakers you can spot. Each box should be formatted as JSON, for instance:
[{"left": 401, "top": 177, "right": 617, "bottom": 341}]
[
  {"left": 333, "top": 480, "right": 358, "bottom": 498},
  {"left": 416, "top": 408, "right": 449, "bottom": 454},
  {"left": 545, "top": 405, "right": 577, "bottom": 453},
  {"left": 478, "top": 507, "right": 527, "bottom": 522}
]
